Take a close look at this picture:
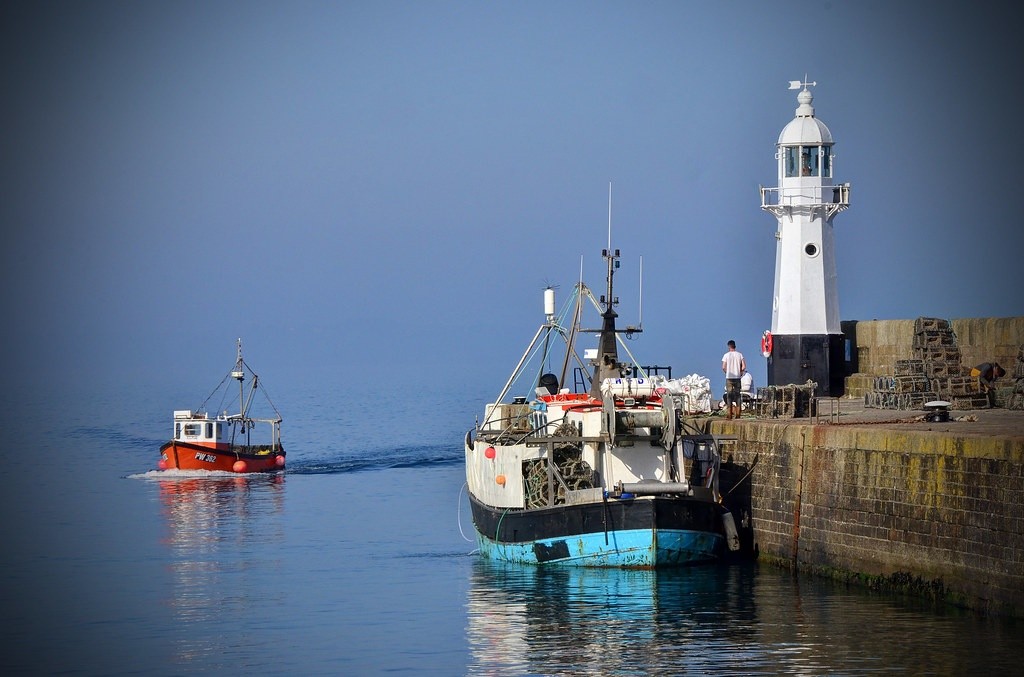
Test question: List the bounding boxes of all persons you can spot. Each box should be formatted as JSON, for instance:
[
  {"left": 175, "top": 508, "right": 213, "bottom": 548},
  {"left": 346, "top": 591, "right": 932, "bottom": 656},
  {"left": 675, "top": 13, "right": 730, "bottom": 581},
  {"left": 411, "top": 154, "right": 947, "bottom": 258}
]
[
  {"left": 721, "top": 341, "right": 745, "bottom": 420},
  {"left": 723, "top": 363, "right": 754, "bottom": 405},
  {"left": 970, "top": 361, "right": 1007, "bottom": 395}
]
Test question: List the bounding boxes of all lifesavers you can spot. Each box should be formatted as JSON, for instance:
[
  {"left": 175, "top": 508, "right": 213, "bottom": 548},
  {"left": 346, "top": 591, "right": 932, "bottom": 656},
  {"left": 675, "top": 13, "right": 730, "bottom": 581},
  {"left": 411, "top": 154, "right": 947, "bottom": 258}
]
[{"left": 761, "top": 330, "right": 773, "bottom": 358}]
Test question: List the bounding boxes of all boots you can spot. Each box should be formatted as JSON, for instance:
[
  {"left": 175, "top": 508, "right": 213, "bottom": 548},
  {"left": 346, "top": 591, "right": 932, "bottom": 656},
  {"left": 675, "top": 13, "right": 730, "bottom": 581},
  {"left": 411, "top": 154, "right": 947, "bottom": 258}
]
[
  {"left": 735, "top": 406, "right": 741, "bottom": 419},
  {"left": 726, "top": 407, "right": 732, "bottom": 420}
]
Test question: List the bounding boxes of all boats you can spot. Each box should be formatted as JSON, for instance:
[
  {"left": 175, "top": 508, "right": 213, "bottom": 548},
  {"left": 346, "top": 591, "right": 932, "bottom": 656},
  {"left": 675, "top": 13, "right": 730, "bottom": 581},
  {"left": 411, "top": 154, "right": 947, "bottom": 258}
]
[
  {"left": 465, "top": 180, "right": 749, "bottom": 569},
  {"left": 160, "top": 338, "right": 288, "bottom": 474}
]
